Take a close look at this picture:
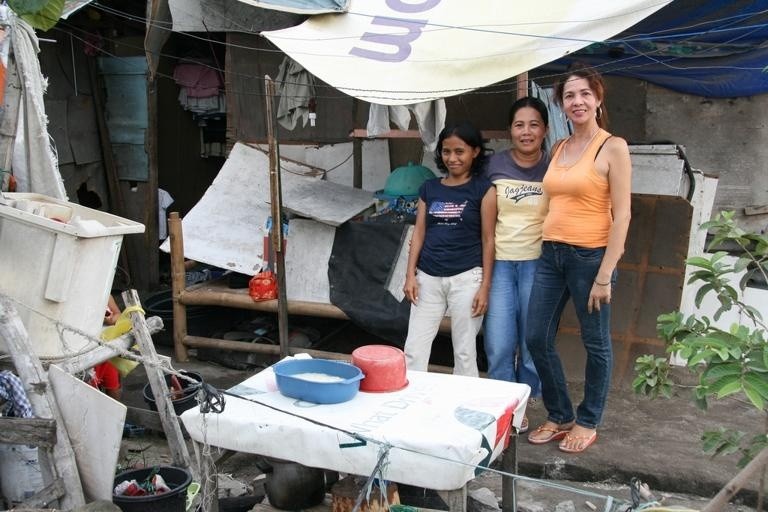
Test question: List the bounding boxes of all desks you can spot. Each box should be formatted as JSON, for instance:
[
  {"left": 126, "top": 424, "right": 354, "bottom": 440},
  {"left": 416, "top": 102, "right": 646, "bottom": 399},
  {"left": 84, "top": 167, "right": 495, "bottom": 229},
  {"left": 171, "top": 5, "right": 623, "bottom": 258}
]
[{"left": 179, "top": 357, "right": 531, "bottom": 512}]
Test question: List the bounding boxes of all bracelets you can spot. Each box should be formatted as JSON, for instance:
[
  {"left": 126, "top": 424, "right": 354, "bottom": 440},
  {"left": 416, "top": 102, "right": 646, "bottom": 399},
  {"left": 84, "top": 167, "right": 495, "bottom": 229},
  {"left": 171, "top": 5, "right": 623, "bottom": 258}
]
[{"left": 594, "top": 279, "right": 611, "bottom": 287}]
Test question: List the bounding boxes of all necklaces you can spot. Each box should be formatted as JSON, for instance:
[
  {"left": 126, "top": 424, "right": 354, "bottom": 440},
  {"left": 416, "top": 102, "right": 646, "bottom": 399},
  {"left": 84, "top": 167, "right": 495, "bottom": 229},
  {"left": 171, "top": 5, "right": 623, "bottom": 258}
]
[
  {"left": 563, "top": 130, "right": 597, "bottom": 164},
  {"left": 515, "top": 146, "right": 541, "bottom": 162}
]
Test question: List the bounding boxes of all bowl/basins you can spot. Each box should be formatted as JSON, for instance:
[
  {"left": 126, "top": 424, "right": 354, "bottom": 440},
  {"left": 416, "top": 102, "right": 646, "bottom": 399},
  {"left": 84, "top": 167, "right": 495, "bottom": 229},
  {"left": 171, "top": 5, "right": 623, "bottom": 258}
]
[
  {"left": 351, "top": 343, "right": 409, "bottom": 392},
  {"left": 270, "top": 358, "right": 365, "bottom": 406}
]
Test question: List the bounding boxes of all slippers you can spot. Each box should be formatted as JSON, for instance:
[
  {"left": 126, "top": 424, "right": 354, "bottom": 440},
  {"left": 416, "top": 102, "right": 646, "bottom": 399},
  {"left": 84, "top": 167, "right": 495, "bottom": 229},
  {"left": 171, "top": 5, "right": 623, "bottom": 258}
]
[
  {"left": 518, "top": 414, "right": 528, "bottom": 433},
  {"left": 528, "top": 423, "right": 571, "bottom": 443},
  {"left": 558, "top": 432, "right": 597, "bottom": 453}
]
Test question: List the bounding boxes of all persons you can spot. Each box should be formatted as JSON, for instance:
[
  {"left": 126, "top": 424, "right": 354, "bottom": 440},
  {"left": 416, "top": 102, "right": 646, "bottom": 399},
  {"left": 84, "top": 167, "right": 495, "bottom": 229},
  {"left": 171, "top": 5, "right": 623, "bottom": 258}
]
[
  {"left": 528, "top": 67, "right": 632, "bottom": 454},
  {"left": 400, "top": 121, "right": 497, "bottom": 378},
  {"left": 482, "top": 96, "right": 554, "bottom": 433}
]
